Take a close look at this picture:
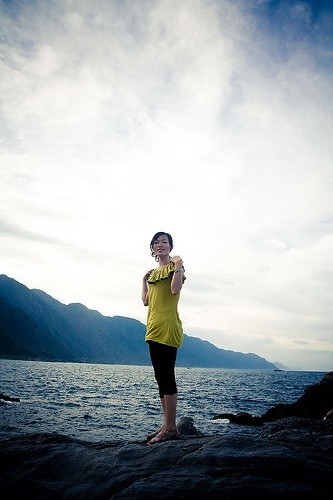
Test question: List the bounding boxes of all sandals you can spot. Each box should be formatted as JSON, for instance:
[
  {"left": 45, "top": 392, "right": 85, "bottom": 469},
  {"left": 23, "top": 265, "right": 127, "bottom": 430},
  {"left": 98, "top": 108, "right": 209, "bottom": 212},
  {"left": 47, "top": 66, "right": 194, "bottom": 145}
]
[
  {"left": 146, "top": 427, "right": 163, "bottom": 440},
  {"left": 146, "top": 430, "right": 179, "bottom": 445}
]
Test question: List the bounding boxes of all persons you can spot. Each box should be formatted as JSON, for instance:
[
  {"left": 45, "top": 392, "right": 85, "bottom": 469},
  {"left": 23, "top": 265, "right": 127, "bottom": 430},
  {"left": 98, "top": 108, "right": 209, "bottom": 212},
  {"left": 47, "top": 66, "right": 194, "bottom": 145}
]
[{"left": 141, "top": 231, "right": 186, "bottom": 444}]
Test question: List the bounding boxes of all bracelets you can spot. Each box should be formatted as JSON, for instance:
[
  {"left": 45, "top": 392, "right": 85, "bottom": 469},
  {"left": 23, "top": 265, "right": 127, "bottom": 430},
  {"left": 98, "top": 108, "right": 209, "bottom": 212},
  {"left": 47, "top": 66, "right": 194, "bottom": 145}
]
[{"left": 174, "top": 268, "right": 185, "bottom": 273}]
